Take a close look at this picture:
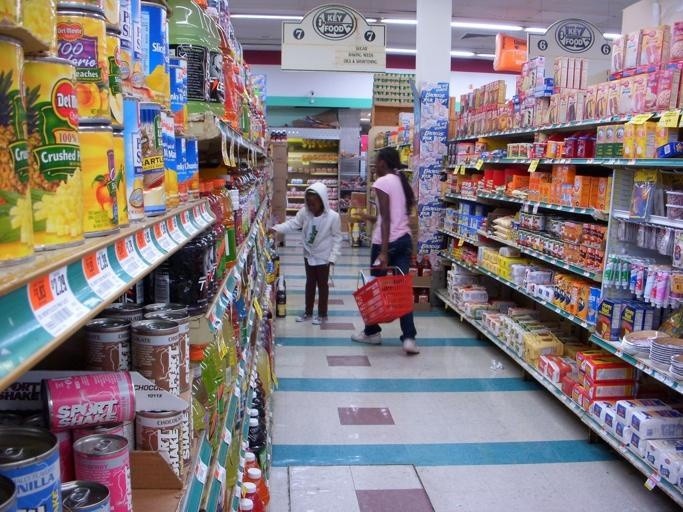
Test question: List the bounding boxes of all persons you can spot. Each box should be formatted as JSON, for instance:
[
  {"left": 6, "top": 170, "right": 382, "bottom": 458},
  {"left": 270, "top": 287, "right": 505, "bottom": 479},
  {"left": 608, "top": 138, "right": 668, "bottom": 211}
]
[
  {"left": 270, "top": 180, "right": 343, "bottom": 325},
  {"left": 352, "top": 146, "right": 421, "bottom": 354}
]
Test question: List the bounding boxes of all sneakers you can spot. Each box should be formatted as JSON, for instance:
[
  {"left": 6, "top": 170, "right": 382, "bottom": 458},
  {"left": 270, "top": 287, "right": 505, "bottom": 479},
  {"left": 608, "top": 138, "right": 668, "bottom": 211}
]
[
  {"left": 351, "top": 329, "right": 382, "bottom": 344},
  {"left": 403, "top": 338, "right": 420, "bottom": 354},
  {"left": 312, "top": 315, "right": 329, "bottom": 324},
  {"left": 294, "top": 313, "right": 313, "bottom": 322}
]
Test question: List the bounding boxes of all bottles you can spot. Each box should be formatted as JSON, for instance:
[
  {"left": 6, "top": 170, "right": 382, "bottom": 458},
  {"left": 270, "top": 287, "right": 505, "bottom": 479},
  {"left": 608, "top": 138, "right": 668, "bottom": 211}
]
[{"left": 162, "top": 0, "right": 287, "bottom": 511}]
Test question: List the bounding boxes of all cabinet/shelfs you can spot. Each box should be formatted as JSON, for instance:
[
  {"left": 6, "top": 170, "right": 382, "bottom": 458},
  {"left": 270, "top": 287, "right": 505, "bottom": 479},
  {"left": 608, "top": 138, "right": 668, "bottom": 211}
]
[
  {"left": 290, "top": 156, "right": 369, "bottom": 221},
  {"left": 2, "top": 106, "right": 289, "bottom": 512},
  {"left": 369, "top": 141, "right": 433, "bottom": 314},
  {"left": 433, "top": 109, "right": 682, "bottom": 512}
]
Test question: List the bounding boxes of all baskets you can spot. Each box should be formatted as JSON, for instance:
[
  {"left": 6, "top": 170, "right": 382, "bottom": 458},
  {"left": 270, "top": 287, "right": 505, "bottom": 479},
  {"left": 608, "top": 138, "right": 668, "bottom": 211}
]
[{"left": 352, "top": 272, "right": 416, "bottom": 326}]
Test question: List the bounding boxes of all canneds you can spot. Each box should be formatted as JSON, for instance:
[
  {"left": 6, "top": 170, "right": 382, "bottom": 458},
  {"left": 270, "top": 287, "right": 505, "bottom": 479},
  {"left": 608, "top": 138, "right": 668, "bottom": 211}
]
[{"left": 0, "top": 302, "right": 195, "bottom": 512}]
